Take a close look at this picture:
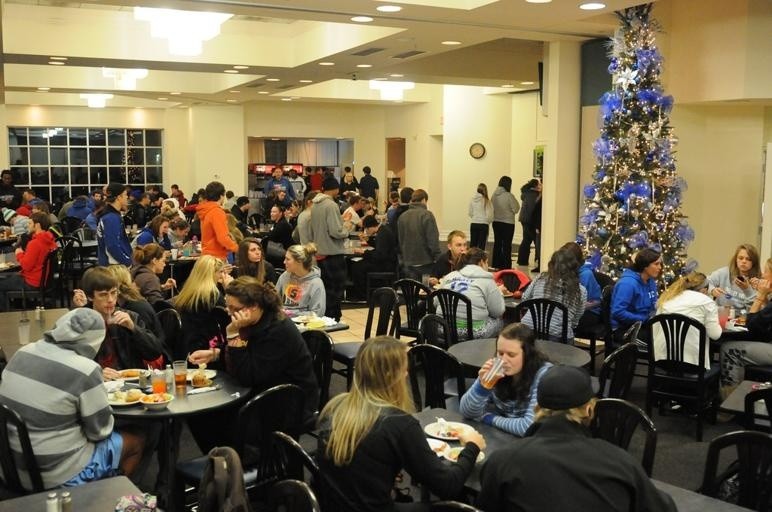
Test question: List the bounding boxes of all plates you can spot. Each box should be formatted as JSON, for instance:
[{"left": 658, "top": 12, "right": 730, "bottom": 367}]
[
  {"left": 423, "top": 436, "right": 450, "bottom": 459},
  {"left": 112, "top": 368, "right": 150, "bottom": 381},
  {"left": 444, "top": 447, "right": 486, "bottom": 464},
  {"left": 289, "top": 312, "right": 335, "bottom": 329},
  {"left": 108, "top": 389, "right": 144, "bottom": 405},
  {"left": 107, "top": 383, "right": 154, "bottom": 393},
  {"left": 186, "top": 368, "right": 216, "bottom": 381},
  {"left": 424, "top": 419, "right": 474, "bottom": 440},
  {"left": 502, "top": 292, "right": 514, "bottom": 297}
]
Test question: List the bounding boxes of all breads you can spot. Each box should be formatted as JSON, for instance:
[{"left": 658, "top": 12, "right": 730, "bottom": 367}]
[
  {"left": 121, "top": 371, "right": 139, "bottom": 377},
  {"left": 191, "top": 373, "right": 209, "bottom": 388},
  {"left": 125, "top": 389, "right": 142, "bottom": 403}
]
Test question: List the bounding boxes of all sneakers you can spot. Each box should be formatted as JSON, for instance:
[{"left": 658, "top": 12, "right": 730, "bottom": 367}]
[
  {"left": 322, "top": 177, "right": 340, "bottom": 191},
  {"left": 107, "top": 183, "right": 124, "bottom": 198}
]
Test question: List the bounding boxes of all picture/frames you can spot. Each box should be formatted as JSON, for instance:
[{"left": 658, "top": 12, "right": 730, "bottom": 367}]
[
  {"left": 469, "top": 142, "right": 486, "bottom": 159},
  {"left": 533, "top": 145, "right": 544, "bottom": 178}
]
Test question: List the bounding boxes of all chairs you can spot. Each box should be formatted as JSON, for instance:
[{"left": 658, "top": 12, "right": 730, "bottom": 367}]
[{"left": 0, "top": 200, "right": 772, "bottom": 512}]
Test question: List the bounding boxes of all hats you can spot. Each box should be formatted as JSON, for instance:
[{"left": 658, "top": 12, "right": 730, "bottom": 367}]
[
  {"left": 2, "top": 208, "right": 16, "bottom": 222},
  {"left": 536, "top": 366, "right": 598, "bottom": 410}
]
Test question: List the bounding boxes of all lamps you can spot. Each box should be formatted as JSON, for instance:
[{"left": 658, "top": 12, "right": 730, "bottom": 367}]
[
  {"left": 101, "top": 68, "right": 148, "bottom": 90},
  {"left": 368, "top": 80, "right": 414, "bottom": 101},
  {"left": 80, "top": 93, "right": 113, "bottom": 108},
  {"left": 133, "top": 7, "right": 234, "bottom": 58}
]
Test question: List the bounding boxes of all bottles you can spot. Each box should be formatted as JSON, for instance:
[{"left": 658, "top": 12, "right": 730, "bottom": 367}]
[
  {"left": 713, "top": 304, "right": 747, "bottom": 327},
  {"left": 166, "top": 364, "right": 173, "bottom": 384},
  {"left": 138, "top": 370, "right": 147, "bottom": 388},
  {"left": 758, "top": 381, "right": 771, "bottom": 403},
  {"left": 35, "top": 306, "right": 46, "bottom": 322},
  {"left": 183, "top": 234, "right": 198, "bottom": 257}
]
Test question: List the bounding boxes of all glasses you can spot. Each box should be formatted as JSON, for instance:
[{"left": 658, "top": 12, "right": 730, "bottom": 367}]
[{"left": 224, "top": 305, "right": 246, "bottom": 316}]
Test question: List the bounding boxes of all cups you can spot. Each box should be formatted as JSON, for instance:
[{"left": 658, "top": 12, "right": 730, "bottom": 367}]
[
  {"left": 127, "top": 223, "right": 138, "bottom": 235},
  {"left": 150, "top": 373, "right": 168, "bottom": 394},
  {"left": 18, "top": 325, "right": 31, "bottom": 345},
  {"left": 479, "top": 356, "right": 508, "bottom": 390},
  {"left": 421, "top": 274, "right": 430, "bottom": 289},
  {"left": 170, "top": 249, "right": 178, "bottom": 260},
  {"left": 173, "top": 360, "right": 187, "bottom": 389},
  {"left": 249, "top": 190, "right": 263, "bottom": 199},
  {"left": 1, "top": 253, "right": 6, "bottom": 269}
]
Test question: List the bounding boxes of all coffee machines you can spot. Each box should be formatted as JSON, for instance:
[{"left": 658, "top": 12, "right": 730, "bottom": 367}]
[
  {"left": 249, "top": 164, "right": 281, "bottom": 199},
  {"left": 281, "top": 164, "right": 304, "bottom": 199}
]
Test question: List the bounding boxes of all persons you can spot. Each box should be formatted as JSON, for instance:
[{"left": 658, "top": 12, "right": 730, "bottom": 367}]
[
  {"left": 307, "top": 334, "right": 486, "bottom": 511},
  {"left": 461, "top": 324, "right": 556, "bottom": 438},
  {"left": 1, "top": 165, "right": 439, "bottom": 324},
  {"left": 479, "top": 363, "right": 678, "bottom": 510},
  {"left": 422, "top": 230, "right": 772, "bottom": 400},
  {"left": 468, "top": 175, "right": 543, "bottom": 272},
  {"left": 0, "top": 265, "right": 318, "bottom": 512}
]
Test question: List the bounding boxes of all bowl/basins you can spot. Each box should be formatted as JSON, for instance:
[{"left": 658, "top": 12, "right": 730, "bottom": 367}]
[{"left": 140, "top": 393, "right": 173, "bottom": 411}]
